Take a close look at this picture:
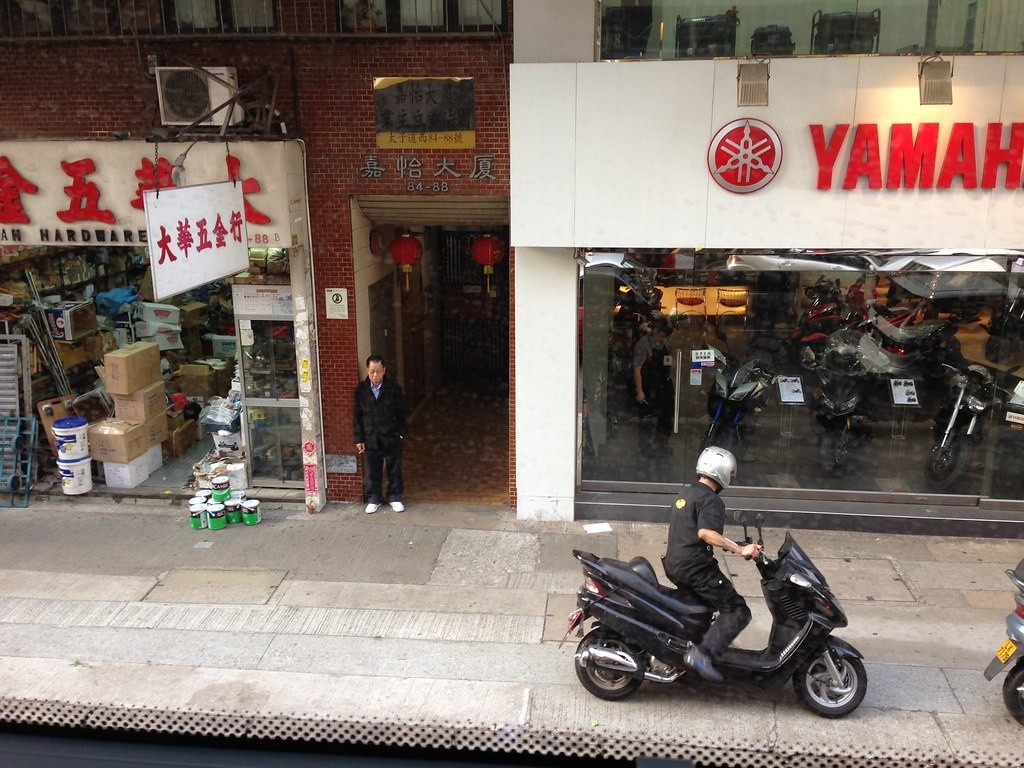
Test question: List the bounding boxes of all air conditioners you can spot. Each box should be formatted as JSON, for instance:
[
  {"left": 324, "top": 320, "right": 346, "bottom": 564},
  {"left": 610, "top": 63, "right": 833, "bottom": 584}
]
[{"left": 155, "top": 66, "right": 243, "bottom": 126}]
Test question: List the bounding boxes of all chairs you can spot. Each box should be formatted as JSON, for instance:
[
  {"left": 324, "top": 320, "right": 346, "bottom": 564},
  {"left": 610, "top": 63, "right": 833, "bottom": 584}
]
[
  {"left": 675, "top": 287, "right": 707, "bottom": 329},
  {"left": 716, "top": 288, "right": 750, "bottom": 328}
]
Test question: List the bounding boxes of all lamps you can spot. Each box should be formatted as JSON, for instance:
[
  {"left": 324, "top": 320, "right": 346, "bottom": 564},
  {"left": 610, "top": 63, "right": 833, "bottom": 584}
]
[
  {"left": 736, "top": 53, "right": 770, "bottom": 108},
  {"left": 918, "top": 48, "right": 952, "bottom": 105}
]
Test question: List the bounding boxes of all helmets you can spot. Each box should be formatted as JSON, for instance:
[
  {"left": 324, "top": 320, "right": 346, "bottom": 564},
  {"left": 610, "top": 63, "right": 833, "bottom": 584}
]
[{"left": 695, "top": 446, "right": 738, "bottom": 489}]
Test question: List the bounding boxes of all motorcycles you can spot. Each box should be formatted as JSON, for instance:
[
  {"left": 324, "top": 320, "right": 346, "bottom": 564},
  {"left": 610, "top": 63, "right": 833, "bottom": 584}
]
[
  {"left": 699, "top": 326, "right": 785, "bottom": 456},
  {"left": 799, "top": 328, "right": 884, "bottom": 475},
  {"left": 922, "top": 363, "right": 1023, "bottom": 492}
]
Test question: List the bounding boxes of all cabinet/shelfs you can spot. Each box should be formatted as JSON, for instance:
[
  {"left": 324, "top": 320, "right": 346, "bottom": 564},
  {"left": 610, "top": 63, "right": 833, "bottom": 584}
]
[{"left": 0, "top": 243, "right": 153, "bottom": 409}]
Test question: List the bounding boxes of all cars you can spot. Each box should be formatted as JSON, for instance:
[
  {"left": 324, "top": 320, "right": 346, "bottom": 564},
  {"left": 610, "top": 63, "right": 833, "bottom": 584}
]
[{"left": 578, "top": 248, "right": 1024, "bottom": 399}]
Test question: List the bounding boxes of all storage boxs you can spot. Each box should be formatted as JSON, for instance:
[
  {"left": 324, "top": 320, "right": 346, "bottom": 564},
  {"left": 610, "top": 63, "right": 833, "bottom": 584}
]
[{"left": 37, "top": 266, "right": 238, "bottom": 488}]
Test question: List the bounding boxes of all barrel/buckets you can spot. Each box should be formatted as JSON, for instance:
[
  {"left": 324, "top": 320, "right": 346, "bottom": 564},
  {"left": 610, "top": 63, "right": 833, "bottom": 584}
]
[
  {"left": 51, "top": 416, "right": 89, "bottom": 461},
  {"left": 187, "top": 476, "right": 262, "bottom": 531},
  {"left": 55, "top": 453, "right": 93, "bottom": 495}
]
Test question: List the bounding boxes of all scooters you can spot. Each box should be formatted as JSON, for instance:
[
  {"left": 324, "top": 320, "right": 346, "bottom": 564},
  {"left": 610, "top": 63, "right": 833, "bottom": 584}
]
[
  {"left": 984, "top": 559, "right": 1024, "bottom": 727},
  {"left": 558, "top": 511, "right": 868, "bottom": 719}
]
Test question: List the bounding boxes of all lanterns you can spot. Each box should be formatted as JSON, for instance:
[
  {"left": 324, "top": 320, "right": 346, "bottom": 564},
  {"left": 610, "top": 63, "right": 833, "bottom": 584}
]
[
  {"left": 389, "top": 232, "right": 423, "bottom": 292},
  {"left": 472, "top": 234, "right": 505, "bottom": 293}
]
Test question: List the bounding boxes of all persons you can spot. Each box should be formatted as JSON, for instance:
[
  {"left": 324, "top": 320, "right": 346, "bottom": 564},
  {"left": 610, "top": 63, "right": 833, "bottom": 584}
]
[
  {"left": 634, "top": 317, "right": 677, "bottom": 459},
  {"left": 354, "top": 356, "right": 407, "bottom": 514},
  {"left": 661, "top": 445, "right": 762, "bottom": 683}
]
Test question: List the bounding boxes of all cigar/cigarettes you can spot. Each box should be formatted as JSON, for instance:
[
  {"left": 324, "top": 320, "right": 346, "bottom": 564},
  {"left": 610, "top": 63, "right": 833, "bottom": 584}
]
[{"left": 358, "top": 449, "right": 365, "bottom": 454}]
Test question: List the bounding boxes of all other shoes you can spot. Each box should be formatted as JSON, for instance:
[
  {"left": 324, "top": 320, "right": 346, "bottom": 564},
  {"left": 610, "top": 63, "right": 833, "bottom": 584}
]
[
  {"left": 639, "top": 441, "right": 655, "bottom": 459},
  {"left": 389, "top": 501, "right": 404, "bottom": 512},
  {"left": 684, "top": 646, "right": 724, "bottom": 683},
  {"left": 365, "top": 503, "right": 381, "bottom": 514}
]
